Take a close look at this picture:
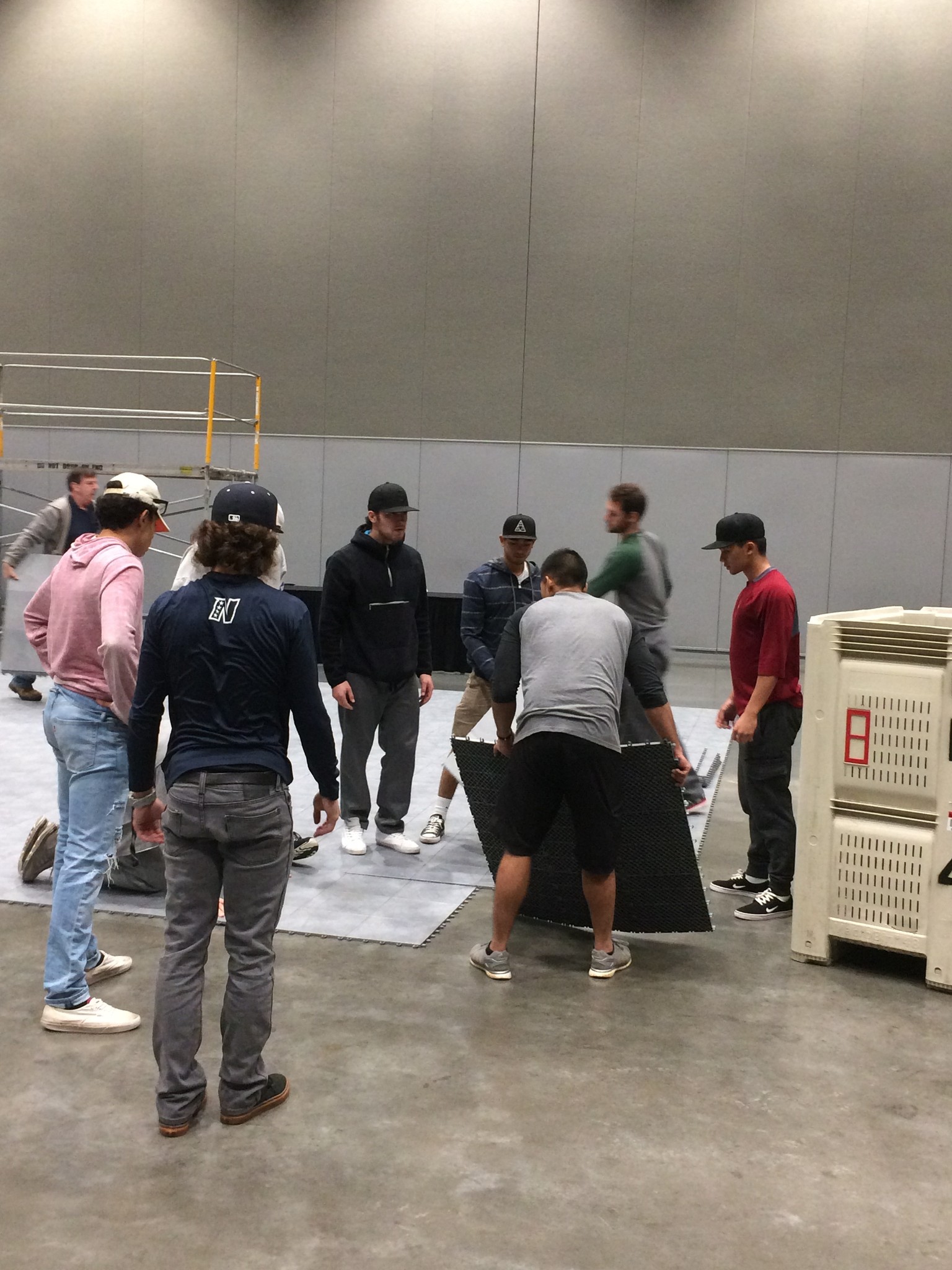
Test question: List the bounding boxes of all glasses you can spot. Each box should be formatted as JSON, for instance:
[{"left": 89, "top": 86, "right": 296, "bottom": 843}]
[{"left": 151, "top": 497, "right": 169, "bottom": 515}]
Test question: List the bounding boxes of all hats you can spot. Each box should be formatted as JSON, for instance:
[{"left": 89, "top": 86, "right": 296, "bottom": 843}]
[
  {"left": 368, "top": 481, "right": 420, "bottom": 512},
  {"left": 210, "top": 481, "right": 285, "bottom": 534},
  {"left": 275, "top": 503, "right": 285, "bottom": 529},
  {"left": 103, "top": 472, "right": 171, "bottom": 532},
  {"left": 501, "top": 514, "right": 538, "bottom": 540},
  {"left": 701, "top": 511, "right": 766, "bottom": 550}
]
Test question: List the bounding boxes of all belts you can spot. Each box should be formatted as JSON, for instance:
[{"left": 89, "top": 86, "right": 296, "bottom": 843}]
[{"left": 176, "top": 770, "right": 285, "bottom": 786}]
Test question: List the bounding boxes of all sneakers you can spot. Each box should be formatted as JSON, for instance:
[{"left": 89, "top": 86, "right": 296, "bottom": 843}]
[
  {"left": 221, "top": 1072, "right": 290, "bottom": 1125},
  {"left": 468, "top": 939, "right": 512, "bottom": 979},
  {"left": 158, "top": 1088, "right": 208, "bottom": 1137},
  {"left": 733, "top": 886, "right": 793, "bottom": 921},
  {"left": 710, "top": 869, "right": 772, "bottom": 899},
  {"left": 588, "top": 937, "right": 632, "bottom": 978},
  {"left": 340, "top": 816, "right": 367, "bottom": 856},
  {"left": 374, "top": 827, "right": 421, "bottom": 854},
  {"left": 40, "top": 997, "right": 142, "bottom": 1034},
  {"left": 17, "top": 815, "right": 61, "bottom": 882},
  {"left": 420, "top": 814, "right": 445, "bottom": 844},
  {"left": 84, "top": 950, "right": 133, "bottom": 986},
  {"left": 291, "top": 830, "right": 319, "bottom": 860}
]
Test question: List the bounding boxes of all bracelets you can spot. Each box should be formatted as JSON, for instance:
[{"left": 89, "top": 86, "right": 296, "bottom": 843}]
[{"left": 496, "top": 727, "right": 513, "bottom": 740}]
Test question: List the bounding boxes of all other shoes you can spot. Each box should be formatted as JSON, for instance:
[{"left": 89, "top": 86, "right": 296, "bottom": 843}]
[
  {"left": 686, "top": 796, "right": 706, "bottom": 813},
  {"left": 8, "top": 678, "right": 42, "bottom": 701}
]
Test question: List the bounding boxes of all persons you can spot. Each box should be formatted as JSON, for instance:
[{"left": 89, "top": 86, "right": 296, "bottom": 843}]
[
  {"left": 466, "top": 549, "right": 694, "bottom": 980},
  {"left": 1, "top": 464, "right": 100, "bottom": 705},
  {"left": 700, "top": 510, "right": 803, "bottom": 919},
  {"left": 125, "top": 482, "right": 341, "bottom": 1138},
  {"left": 311, "top": 480, "right": 437, "bottom": 855},
  {"left": 23, "top": 475, "right": 170, "bottom": 1033},
  {"left": 18, "top": 480, "right": 318, "bottom": 884},
  {"left": 419, "top": 484, "right": 706, "bottom": 844}
]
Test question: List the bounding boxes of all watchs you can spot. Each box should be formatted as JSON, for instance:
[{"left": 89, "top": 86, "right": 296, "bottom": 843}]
[{"left": 127, "top": 789, "right": 159, "bottom": 810}]
